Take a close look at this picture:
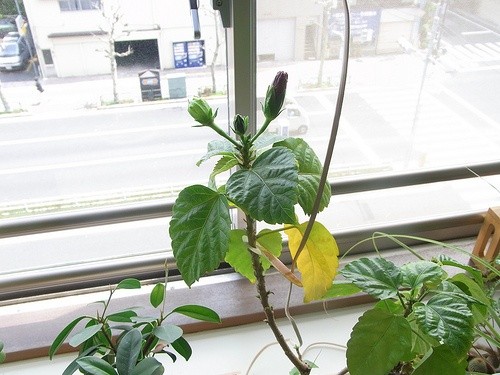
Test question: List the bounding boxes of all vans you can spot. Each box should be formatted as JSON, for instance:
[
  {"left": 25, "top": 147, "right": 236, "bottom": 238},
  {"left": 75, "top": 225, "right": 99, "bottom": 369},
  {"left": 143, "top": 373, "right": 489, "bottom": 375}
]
[
  {"left": 257, "top": 102, "right": 308, "bottom": 134},
  {"left": 0, "top": 30, "right": 29, "bottom": 72}
]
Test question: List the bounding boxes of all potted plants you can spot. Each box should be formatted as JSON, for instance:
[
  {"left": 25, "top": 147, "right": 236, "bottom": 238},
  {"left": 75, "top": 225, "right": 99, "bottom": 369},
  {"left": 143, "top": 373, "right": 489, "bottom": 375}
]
[
  {"left": 46, "top": 278, "right": 222, "bottom": 375},
  {"left": 168, "top": 71, "right": 500, "bottom": 375}
]
